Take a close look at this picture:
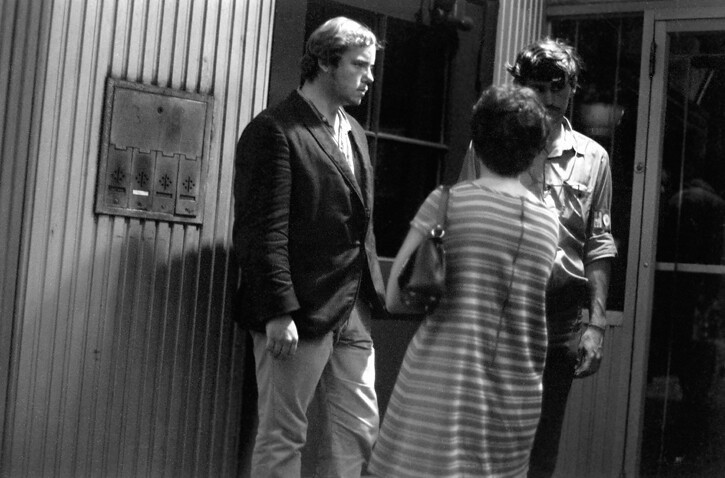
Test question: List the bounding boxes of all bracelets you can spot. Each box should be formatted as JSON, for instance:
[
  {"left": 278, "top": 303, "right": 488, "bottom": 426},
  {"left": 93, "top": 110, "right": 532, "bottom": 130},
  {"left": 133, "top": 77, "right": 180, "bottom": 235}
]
[{"left": 586, "top": 322, "right": 606, "bottom": 333}]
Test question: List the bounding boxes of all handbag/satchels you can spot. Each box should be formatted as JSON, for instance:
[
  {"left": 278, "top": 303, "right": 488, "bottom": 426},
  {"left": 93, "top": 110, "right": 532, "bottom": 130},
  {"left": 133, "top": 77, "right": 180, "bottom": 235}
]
[{"left": 398, "top": 184, "right": 451, "bottom": 310}]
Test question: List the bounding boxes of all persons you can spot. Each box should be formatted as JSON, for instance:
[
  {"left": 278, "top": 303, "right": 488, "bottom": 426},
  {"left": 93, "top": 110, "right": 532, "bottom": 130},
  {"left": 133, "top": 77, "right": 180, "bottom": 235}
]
[
  {"left": 457, "top": 37, "right": 617, "bottom": 478},
  {"left": 386, "top": 85, "right": 548, "bottom": 478},
  {"left": 231, "top": 16, "right": 386, "bottom": 478}
]
